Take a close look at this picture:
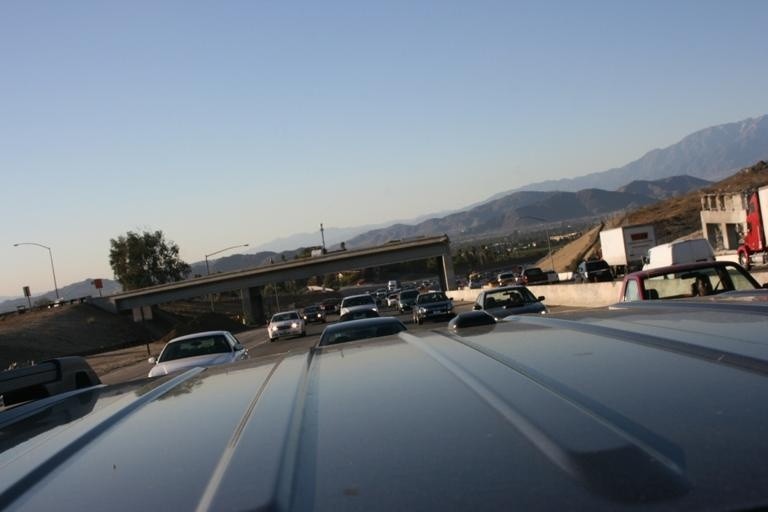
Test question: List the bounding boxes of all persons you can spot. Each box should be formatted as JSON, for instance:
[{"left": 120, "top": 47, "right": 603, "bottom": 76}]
[{"left": 693, "top": 274, "right": 713, "bottom": 297}]
